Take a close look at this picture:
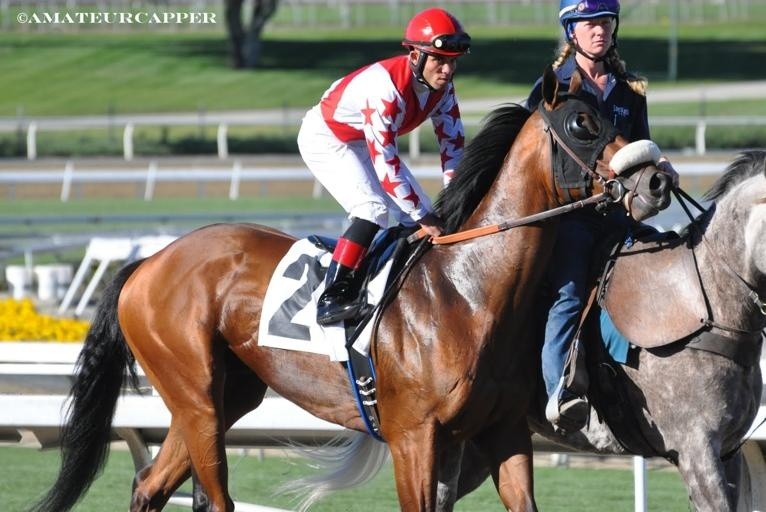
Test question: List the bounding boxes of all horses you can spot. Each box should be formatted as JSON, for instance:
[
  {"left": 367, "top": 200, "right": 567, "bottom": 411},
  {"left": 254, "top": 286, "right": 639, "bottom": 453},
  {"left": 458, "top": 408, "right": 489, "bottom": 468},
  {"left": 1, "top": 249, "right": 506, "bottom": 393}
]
[
  {"left": 264, "top": 146, "right": 766, "bottom": 511},
  {"left": 26, "top": 61, "right": 674, "bottom": 512}
]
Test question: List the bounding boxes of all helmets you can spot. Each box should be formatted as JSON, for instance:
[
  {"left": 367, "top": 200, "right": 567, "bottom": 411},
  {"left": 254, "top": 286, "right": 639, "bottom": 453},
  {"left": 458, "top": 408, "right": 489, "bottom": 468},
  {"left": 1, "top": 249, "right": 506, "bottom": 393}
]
[
  {"left": 559, "top": 0, "right": 619, "bottom": 25},
  {"left": 403, "top": 9, "right": 471, "bottom": 56}
]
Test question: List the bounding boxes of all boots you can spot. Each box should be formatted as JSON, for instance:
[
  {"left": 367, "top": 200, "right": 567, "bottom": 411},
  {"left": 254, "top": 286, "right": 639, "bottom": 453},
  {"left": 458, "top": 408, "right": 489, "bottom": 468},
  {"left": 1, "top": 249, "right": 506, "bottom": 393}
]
[{"left": 317, "top": 237, "right": 368, "bottom": 323}]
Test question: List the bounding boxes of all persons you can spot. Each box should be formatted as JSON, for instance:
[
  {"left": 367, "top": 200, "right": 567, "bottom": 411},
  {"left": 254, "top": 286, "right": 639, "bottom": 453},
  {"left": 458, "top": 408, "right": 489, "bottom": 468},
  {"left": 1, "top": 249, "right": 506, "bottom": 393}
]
[
  {"left": 295, "top": 4, "right": 468, "bottom": 328},
  {"left": 523, "top": 0, "right": 683, "bottom": 433}
]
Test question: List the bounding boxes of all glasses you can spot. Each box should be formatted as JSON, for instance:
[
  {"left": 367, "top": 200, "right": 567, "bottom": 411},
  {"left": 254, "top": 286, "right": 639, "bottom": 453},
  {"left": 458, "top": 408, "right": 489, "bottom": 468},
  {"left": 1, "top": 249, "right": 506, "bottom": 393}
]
[
  {"left": 560, "top": 0, "right": 621, "bottom": 16},
  {"left": 402, "top": 32, "right": 470, "bottom": 53}
]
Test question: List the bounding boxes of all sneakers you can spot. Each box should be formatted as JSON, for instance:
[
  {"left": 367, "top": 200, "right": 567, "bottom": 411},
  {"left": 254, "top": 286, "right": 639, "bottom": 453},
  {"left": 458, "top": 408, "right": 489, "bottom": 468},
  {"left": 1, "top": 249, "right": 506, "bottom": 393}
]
[{"left": 552, "top": 398, "right": 589, "bottom": 432}]
